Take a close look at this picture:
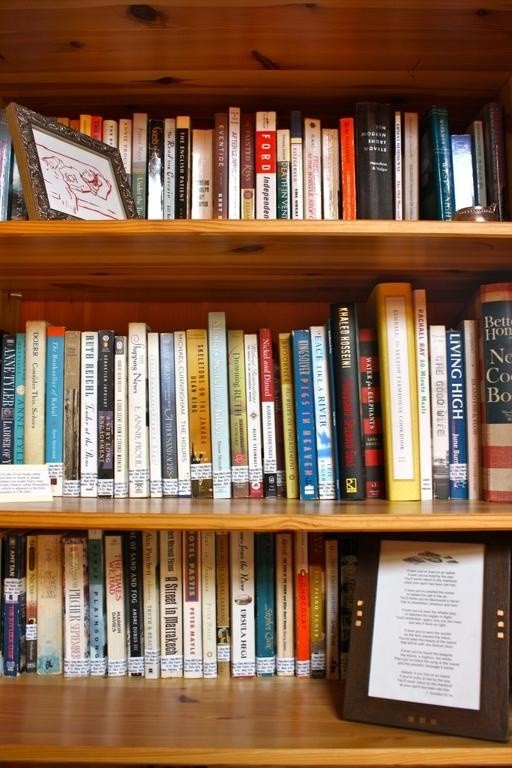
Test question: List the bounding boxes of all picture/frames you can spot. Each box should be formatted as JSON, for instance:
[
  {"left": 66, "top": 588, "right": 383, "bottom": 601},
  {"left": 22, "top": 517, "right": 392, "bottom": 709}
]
[
  {"left": 337, "top": 533, "right": 510, "bottom": 742},
  {"left": 6, "top": 102, "right": 143, "bottom": 221}
]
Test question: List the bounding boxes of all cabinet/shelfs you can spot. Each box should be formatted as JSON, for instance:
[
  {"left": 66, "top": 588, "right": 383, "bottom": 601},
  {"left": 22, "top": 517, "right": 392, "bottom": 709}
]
[{"left": 0, "top": 0, "right": 512, "bottom": 768}]
